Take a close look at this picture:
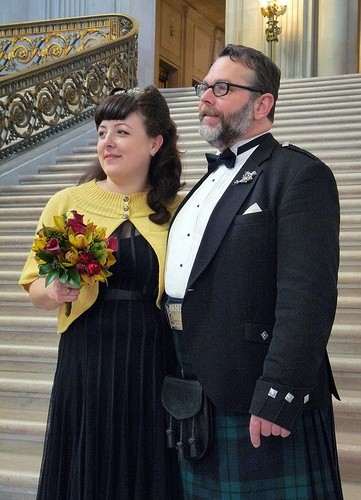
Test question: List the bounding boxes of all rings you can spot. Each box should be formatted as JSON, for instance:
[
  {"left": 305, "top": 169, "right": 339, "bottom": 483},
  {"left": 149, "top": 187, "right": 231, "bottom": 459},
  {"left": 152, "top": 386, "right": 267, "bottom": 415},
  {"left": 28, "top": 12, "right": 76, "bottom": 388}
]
[{"left": 65, "top": 288, "right": 71, "bottom": 294}]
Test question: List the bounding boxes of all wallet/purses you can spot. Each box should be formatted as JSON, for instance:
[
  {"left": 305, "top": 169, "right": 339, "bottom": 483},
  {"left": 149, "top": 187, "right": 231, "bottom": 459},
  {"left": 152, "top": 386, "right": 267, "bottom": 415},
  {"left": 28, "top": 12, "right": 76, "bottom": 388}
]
[{"left": 160, "top": 375, "right": 213, "bottom": 462}]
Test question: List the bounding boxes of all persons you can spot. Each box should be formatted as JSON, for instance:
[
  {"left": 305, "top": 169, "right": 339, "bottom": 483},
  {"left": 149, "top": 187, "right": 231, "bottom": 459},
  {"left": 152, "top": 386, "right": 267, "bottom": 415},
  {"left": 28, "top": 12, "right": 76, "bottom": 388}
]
[
  {"left": 17, "top": 85, "right": 185, "bottom": 500},
  {"left": 164, "top": 43, "right": 345, "bottom": 500}
]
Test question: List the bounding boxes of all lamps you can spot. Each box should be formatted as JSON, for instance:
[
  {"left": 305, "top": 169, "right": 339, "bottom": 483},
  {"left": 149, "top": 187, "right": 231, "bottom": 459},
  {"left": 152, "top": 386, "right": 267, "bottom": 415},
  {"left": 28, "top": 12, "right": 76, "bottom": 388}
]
[{"left": 261, "top": 0, "right": 287, "bottom": 64}]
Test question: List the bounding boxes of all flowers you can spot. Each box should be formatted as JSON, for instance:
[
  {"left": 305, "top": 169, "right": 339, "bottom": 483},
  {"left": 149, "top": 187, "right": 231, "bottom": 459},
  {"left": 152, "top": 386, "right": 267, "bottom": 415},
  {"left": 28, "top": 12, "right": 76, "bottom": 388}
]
[{"left": 30, "top": 211, "right": 118, "bottom": 317}]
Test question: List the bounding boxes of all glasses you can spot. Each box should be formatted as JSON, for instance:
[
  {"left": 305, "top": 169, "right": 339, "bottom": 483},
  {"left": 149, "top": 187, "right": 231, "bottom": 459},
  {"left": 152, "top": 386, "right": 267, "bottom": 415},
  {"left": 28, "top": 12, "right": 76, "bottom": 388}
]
[{"left": 194, "top": 81, "right": 265, "bottom": 97}]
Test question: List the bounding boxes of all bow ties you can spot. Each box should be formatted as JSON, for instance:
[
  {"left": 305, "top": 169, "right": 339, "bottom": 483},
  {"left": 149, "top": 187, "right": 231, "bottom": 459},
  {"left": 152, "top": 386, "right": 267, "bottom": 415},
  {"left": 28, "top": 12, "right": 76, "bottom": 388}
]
[{"left": 205, "top": 147, "right": 236, "bottom": 174}]
